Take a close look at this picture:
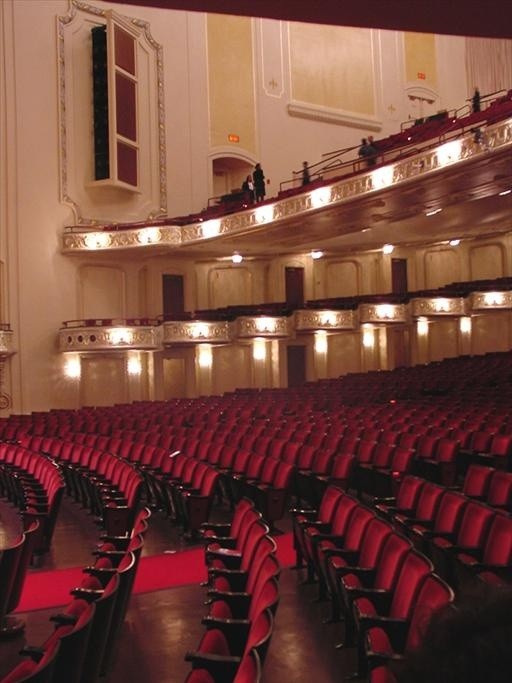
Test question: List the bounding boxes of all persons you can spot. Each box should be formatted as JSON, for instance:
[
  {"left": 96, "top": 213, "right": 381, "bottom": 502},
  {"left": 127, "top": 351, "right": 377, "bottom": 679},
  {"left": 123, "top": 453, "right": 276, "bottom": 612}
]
[
  {"left": 303, "top": 161, "right": 310, "bottom": 184},
  {"left": 473, "top": 86, "right": 480, "bottom": 112},
  {"left": 243, "top": 163, "right": 265, "bottom": 204},
  {"left": 358, "top": 135, "right": 378, "bottom": 166}
]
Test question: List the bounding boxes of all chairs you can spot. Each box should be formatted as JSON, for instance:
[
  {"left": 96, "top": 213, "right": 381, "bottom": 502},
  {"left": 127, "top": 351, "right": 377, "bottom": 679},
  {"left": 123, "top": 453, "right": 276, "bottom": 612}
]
[
  {"left": 180, "top": 494, "right": 281, "bottom": 683},
  {"left": 2, "top": 348, "right": 512, "bottom": 564},
  {"left": 290, "top": 464, "right": 512, "bottom": 683},
  {"left": 1, "top": 505, "right": 156, "bottom": 683}
]
